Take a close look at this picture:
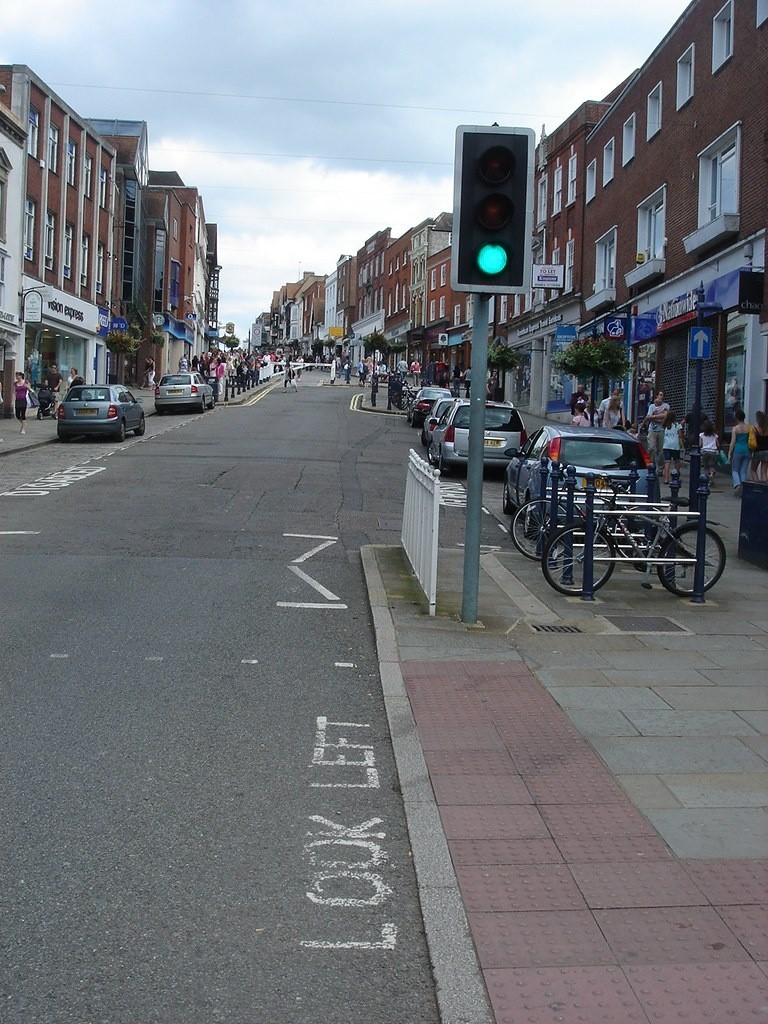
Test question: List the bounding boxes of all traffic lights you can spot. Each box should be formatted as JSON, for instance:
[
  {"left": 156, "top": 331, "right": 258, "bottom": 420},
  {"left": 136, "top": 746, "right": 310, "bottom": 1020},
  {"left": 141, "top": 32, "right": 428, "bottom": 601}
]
[{"left": 449, "top": 125, "right": 535, "bottom": 296}]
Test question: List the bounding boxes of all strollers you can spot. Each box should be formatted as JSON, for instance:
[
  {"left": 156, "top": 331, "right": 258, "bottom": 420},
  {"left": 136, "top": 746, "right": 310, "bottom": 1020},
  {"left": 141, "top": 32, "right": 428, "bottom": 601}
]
[{"left": 36, "top": 389, "right": 58, "bottom": 421}]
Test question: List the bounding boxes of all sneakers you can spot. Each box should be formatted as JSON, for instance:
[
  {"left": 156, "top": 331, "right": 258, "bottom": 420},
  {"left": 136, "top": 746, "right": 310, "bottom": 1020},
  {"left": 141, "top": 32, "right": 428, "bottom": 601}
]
[{"left": 21, "top": 429, "right": 25, "bottom": 434}]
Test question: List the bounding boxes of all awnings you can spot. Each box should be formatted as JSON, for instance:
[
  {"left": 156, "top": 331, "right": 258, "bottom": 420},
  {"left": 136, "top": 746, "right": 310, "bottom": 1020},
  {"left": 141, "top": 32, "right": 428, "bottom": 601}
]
[
  {"left": 411, "top": 318, "right": 449, "bottom": 342},
  {"left": 387, "top": 325, "right": 425, "bottom": 341}
]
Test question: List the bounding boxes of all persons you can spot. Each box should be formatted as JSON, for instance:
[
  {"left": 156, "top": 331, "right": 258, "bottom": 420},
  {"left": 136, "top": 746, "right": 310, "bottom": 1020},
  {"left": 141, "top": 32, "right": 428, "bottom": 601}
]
[
  {"left": 138, "top": 356, "right": 156, "bottom": 391},
  {"left": 282, "top": 354, "right": 351, "bottom": 392},
  {"left": 358, "top": 357, "right": 421, "bottom": 387},
  {"left": 728, "top": 378, "right": 741, "bottom": 402},
  {"left": 439, "top": 358, "right": 500, "bottom": 401},
  {"left": 46, "top": 365, "right": 84, "bottom": 419},
  {"left": 0, "top": 382, "right": 3, "bottom": 443},
  {"left": 571, "top": 384, "right": 768, "bottom": 495},
  {"left": 11, "top": 372, "right": 31, "bottom": 435},
  {"left": 179, "top": 349, "right": 284, "bottom": 396}
]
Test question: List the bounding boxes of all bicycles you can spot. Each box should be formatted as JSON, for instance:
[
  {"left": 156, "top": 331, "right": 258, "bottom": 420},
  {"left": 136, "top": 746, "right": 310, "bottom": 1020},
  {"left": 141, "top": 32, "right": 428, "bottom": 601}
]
[
  {"left": 391, "top": 387, "right": 417, "bottom": 410},
  {"left": 509, "top": 460, "right": 671, "bottom": 563},
  {"left": 539, "top": 475, "right": 726, "bottom": 597}
]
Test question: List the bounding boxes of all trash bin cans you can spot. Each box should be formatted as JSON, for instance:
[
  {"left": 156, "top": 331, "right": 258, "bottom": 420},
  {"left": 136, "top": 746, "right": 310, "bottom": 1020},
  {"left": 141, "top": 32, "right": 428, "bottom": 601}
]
[
  {"left": 737, "top": 480, "right": 768, "bottom": 570},
  {"left": 492, "top": 387, "right": 504, "bottom": 402},
  {"left": 204, "top": 376, "right": 218, "bottom": 402}
]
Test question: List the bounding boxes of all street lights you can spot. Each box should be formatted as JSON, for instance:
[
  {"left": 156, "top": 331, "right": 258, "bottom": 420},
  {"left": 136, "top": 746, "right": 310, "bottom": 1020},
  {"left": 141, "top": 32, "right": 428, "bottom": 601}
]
[{"left": 370, "top": 325, "right": 378, "bottom": 407}]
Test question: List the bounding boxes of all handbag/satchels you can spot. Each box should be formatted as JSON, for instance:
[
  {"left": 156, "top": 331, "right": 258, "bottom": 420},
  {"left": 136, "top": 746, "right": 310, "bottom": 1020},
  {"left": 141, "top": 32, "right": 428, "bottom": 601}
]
[
  {"left": 747, "top": 425, "right": 757, "bottom": 450},
  {"left": 618, "top": 408, "right": 623, "bottom": 425},
  {"left": 29, "top": 392, "right": 41, "bottom": 408},
  {"left": 288, "top": 368, "right": 296, "bottom": 378},
  {"left": 717, "top": 450, "right": 728, "bottom": 467},
  {"left": 291, "top": 379, "right": 297, "bottom": 389}
]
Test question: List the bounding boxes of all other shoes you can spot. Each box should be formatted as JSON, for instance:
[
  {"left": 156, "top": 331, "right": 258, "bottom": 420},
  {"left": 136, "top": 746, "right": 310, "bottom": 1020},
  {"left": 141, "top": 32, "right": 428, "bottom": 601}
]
[
  {"left": 708, "top": 474, "right": 714, "bottom": 485},
  {"left": 734, "top": 486, "right": 741, "bottom": 495}
]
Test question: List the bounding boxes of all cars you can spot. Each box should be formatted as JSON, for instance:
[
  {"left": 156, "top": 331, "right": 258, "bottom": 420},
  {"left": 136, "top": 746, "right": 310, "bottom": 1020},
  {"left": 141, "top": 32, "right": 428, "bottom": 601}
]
[
  {"left": 502, "top": 425, "right": 660, "bottom": 540},
  {"left": 421, "top": 397, "right": 459, "bottom": 449},
  {"left": 154, "top": 373, "right": 214, "bottom": 415},
  {"left": 57, "top": 385, "right": 146, "bottom": 443},
  {"left": 426, "top": 398, "right": 528, "bottom": 478},
  {"left": 407, "top": 388, "right": 452, "bottom": 429}
]
